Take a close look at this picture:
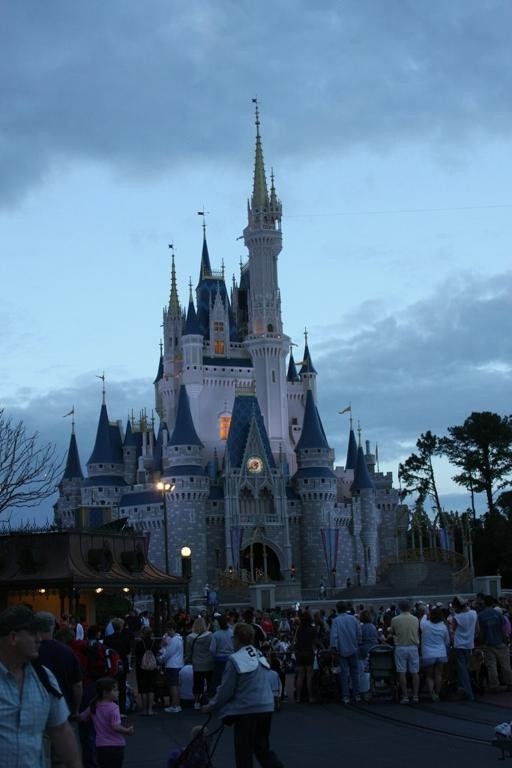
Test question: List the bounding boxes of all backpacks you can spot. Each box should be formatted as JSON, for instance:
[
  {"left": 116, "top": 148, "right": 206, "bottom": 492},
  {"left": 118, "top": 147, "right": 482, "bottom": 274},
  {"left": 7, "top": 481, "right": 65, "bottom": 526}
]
[
  {"left": 140, "top": 640, "right": 157, "bottom": 670},
  {"left": 86, "top": 640, "right": 119, "bottom": 678}
]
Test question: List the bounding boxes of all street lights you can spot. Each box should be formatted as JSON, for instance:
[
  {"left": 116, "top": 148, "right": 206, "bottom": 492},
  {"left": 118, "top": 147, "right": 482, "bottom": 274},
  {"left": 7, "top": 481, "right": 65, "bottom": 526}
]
[
  {"left": 156, "top": 480, "right": 178, "bottom": 573},
  {"left": 180, "top": 546, "right": 194, "bottom": 612}
]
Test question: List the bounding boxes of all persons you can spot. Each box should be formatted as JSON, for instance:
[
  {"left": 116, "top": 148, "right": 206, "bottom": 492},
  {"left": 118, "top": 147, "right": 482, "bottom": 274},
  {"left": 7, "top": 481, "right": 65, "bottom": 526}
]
[
  {"left": 1, "top": 606, "right": 83, "bottom": 767},
  {"left": 202, "top": 622, "right": 283, "bottom": 766},
  {"left": 126, "top": 607, "right": 240, "bottom": 715},
  {"left": 203, "top": 583, "right": 210, "bottom": 598},
  {"left": 319, "top": 585, "right": 325, "bottom": 600},
  {"left": 86, "top": 618, "right": 132, "bottom": 719},
  {"left": 74, "top": 679, "right": 135, "bottom": 767},
  {"left": 31, "top": 612, "right": 84, "bottom": 767},
  {"left": 54, "top": 613, "right": 84, "bottom": 641},
  {"left": 244, "top": 591, "right": 511, "bottom": 711},
  {"left": 55, "top": 628, "right": 106, "bottom": 766}
]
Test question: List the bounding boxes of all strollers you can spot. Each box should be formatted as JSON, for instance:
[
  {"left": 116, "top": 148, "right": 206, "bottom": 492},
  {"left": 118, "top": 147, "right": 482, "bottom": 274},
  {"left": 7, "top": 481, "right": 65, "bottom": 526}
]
[
  {"left": 145, "top": 652, "right": 173, "bottom": 709},
  {"left": 314, "top": 647, "right": 345, "bottom": 703},
  {"left": 270, "top": 649, "right": 290, "bottom": 707},
  {"left": 169, "top": 711, "right": 229, "bottom": 768},
  {"left": 368, "top": 643, "right": 400, "bottom": 704}
]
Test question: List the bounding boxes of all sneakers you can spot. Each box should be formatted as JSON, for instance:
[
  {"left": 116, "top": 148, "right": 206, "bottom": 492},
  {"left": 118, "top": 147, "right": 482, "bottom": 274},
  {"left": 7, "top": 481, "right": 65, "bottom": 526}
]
[
  {"left": 411, "top": 696, "right": 419, "bottom": 703},
  {"left": 164, "top": 706, "right": 179, "bottom": 711},
  {"left": 177, "top": 705, "right": 182, "bottom": 711},
  {"left": 194, "top": 702, "right": 200, "bottom": 709},
  {"left": 400, "top": 696, "right": 409, "bottom": 704}
]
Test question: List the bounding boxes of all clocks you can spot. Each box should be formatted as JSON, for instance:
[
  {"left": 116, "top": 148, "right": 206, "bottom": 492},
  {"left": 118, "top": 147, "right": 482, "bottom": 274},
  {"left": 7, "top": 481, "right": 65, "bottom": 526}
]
[{"left": 247, "top": 456, "right": 264, "bottom": 474}]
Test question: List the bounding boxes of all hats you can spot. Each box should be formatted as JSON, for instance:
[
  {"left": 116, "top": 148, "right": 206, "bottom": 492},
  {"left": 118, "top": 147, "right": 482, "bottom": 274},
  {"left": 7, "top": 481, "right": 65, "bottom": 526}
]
[{"left": 0, "top": 605, "right": 50, "bottom": 636}]
